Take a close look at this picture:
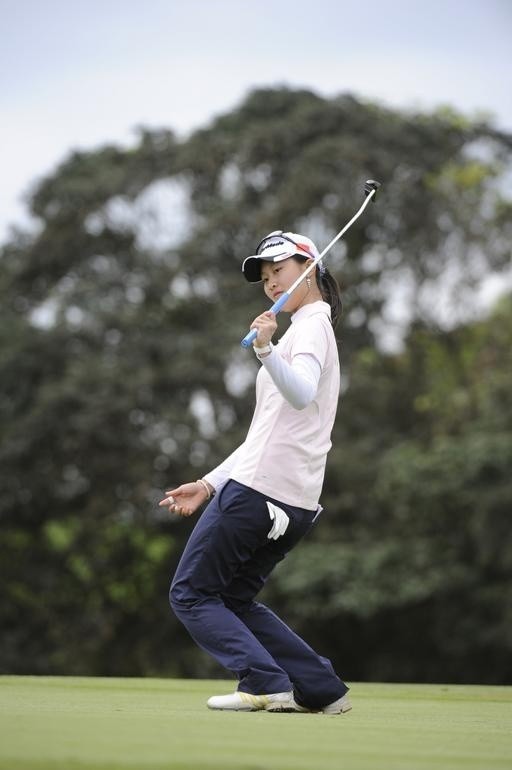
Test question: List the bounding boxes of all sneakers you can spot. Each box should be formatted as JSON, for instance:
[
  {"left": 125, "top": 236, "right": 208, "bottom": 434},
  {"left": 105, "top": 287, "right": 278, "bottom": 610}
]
[{"left": 207, "top": 691, "right": 352, "bottom": 714}]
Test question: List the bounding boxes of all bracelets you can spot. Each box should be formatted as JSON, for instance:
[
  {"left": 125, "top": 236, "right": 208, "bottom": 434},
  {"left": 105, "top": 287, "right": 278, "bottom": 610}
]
[
  {"left": 251, "top": 342, "right": 273, "bottom": 356},
  {"left": 196, "top": 479, "right": 211, "bottom": 502}
]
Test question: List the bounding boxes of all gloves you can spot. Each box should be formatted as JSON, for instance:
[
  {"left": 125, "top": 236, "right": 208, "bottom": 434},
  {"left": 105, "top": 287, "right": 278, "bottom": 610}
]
[{"left": 266, "top": 501, "right": 289, "bottom": 540}]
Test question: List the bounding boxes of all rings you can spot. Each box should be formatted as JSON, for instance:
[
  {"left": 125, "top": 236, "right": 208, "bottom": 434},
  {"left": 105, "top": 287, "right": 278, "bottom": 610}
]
[{"left": 167, "top": 495, "right": 175, "bottom": 504}]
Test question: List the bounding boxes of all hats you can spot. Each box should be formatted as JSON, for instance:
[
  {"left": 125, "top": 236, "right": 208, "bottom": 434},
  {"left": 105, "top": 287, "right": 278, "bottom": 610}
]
[{"left": 242, "top": 230, "right": 322, "bottom": 283}]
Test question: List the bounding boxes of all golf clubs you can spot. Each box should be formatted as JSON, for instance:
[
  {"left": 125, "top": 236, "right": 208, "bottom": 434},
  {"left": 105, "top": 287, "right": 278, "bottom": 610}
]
[{"left": 239, "top": 181, "right": 380, "bottom": 352}]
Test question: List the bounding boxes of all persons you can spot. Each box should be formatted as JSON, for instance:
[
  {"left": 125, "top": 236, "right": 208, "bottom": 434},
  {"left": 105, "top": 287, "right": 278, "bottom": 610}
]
[{"left": 154, "top": 227, "right": 353, "bottom": 719}]
